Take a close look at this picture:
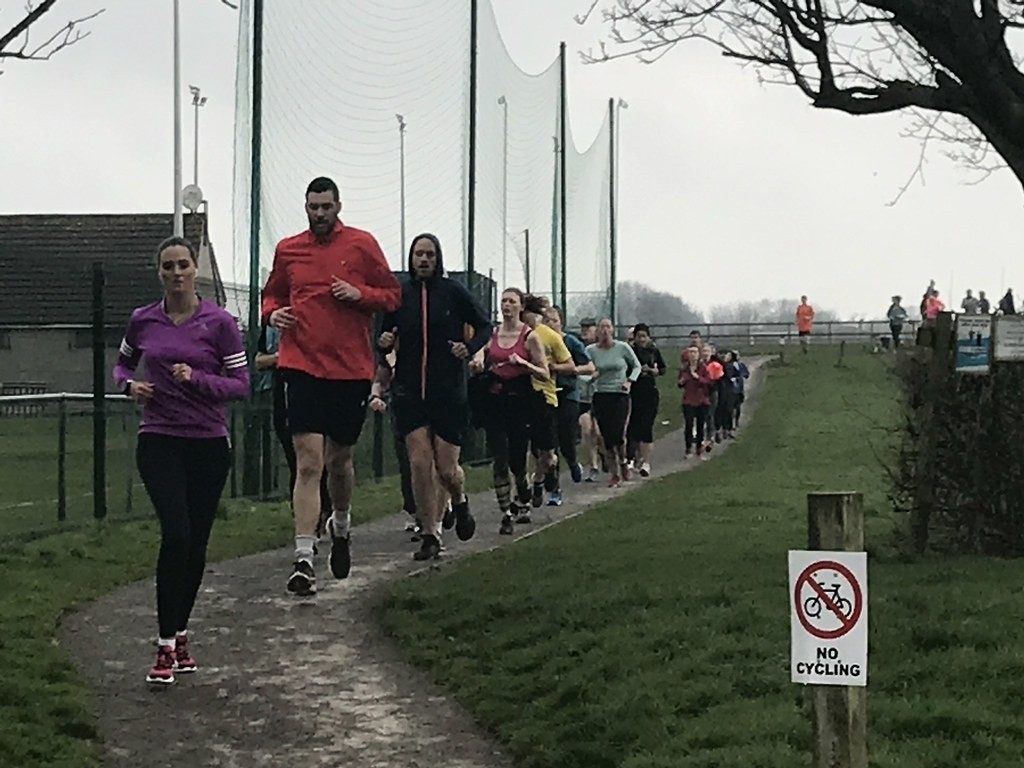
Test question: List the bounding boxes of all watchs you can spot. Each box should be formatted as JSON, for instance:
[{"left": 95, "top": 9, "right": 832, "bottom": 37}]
[{"left": 368, "top": 395, "right": 384, "bottom": 403}]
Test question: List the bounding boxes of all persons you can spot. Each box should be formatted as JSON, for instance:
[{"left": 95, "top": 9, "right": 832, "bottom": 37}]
[
  {"left": 677, "top": 329, "right": 750, "bottom": 458},
  {"left": 1003, "top": 289, "right": 1017, "bottom": 315},
  {"left": 887, "top": 296, "right": 908, "bottom": 348},
  {"left": 113, "top": 238, "right": 251, "bottom": 687},
  {"left": 472, "top": 286, "right": 667, "bottom": 534},
  {"left": 920, "top": 279, "right": 945, "bottom": 319},
  {"left": 362, "top": 235, "right": 491, "bottom": 560},
  {"left": 255, "top": 177, "right": 404, "bottom": 596},
  {"left": 961, "top": 289, "right": 979, "bottom": 314},
  {"left": 978, "top": 291, "right": 989, "bottom": 313},
  {"left": 796, "top": 296, "right": 815, "bottom": 354}
]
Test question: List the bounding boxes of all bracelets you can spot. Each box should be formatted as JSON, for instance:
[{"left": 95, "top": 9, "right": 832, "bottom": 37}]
[{"left": 123, "top": 380, "right": 132, "bottom": 396}]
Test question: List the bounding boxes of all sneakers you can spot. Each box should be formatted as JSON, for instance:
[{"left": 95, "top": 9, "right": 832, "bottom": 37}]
[
  {"left": 176, "top": 634, "right": 198, "bottom": 673},
  {"left": 287, "top": 560, "right": 317, "bottom": 596},
  {"left": 402, "top": 428, "right": 734, "bottom": 561},
  {"left": 146, "top": 644, "right": 179, "bottom": 683},
  {"left": 328, "top": 512, "right": 351, "bottom": 579}
]
